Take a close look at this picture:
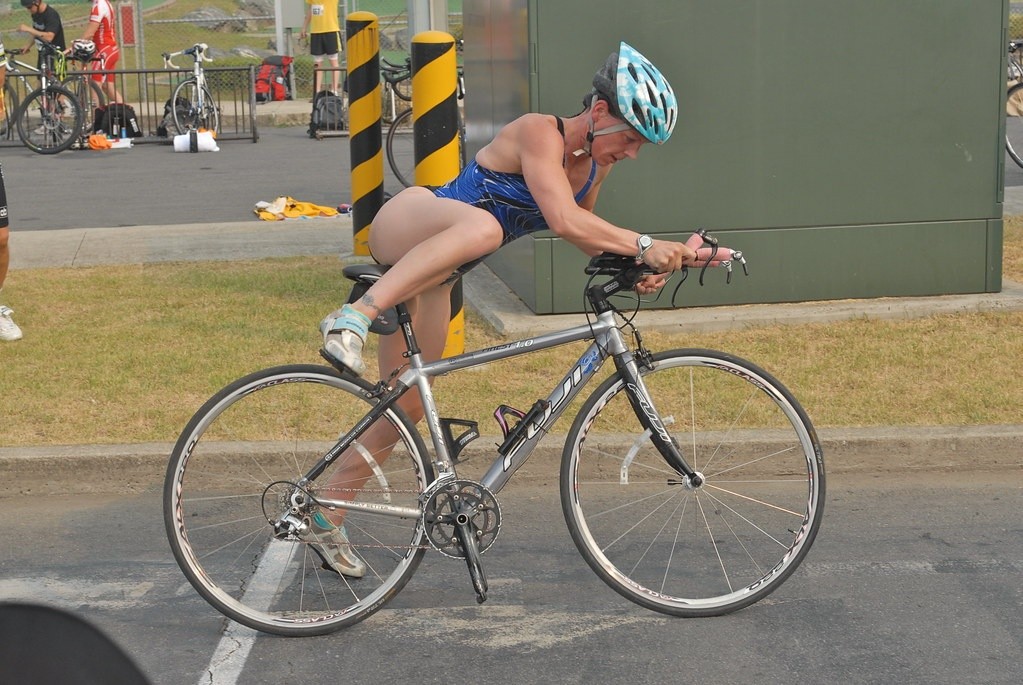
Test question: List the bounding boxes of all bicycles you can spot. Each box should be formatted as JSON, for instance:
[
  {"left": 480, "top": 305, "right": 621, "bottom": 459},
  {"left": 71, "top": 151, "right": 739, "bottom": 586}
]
[
  {"left": 162, "top": 227, "right": 828, "bottom": 640},
  {"left": 53, "top": 39, "right": 107, "bottom": 144},
  {"left": 0, "top": 33, "right": 85, "bottom": 155},
  {"left": 160, "top": 41, "right": 225, "bottom": 140},
  {"left": 1004, "top": 35, "right": 1022, "bottom": 167},
  {"left": 379, "top": 35, "right": 468, "bottom": 191}
]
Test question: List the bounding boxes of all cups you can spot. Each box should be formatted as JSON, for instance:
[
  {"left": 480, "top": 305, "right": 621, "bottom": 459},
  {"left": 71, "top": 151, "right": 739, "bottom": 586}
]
[{"left": 121, "top": 127, "right": 127, "bottom": 138}]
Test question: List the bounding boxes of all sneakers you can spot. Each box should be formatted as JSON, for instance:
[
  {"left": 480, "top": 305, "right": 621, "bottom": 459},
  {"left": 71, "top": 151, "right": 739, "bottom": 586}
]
[
  {"left": 318, "top": 302, "right": 369, "bottom": 378},
  {"left": 0, "top": 304, "right": 23, "bottom": 341},
  {"left": 278, "top": 506, "right": 367, "bottom": 578}
]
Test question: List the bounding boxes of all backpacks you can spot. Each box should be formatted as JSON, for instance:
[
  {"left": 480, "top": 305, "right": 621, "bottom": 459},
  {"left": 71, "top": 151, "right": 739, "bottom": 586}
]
[
  {"left": 163, "top": 94, "right": 191, "bottom": 116},
  {"left": 254, "top": 53, "right": 294, "bottom": 103},
  {"left": 305, "top": 89, "right": 344, "bottom": 138},
  {"left": 154, "top": 106, "right": 203, "bottom": 136},
  {"left": 93, "top": 102, "right": 143, "bottom": 137}
]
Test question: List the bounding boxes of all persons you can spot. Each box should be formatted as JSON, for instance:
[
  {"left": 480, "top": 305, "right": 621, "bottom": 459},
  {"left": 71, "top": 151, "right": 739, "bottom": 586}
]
[
  {"left": 300, "top": 0, "right": 344, "bottom": 102},
  {"left": 16, "top": 0, "right": 67, "bottom": 135},
  {"left": 0, "top": 38, "right": 24, "bottom": 342},
  {"left": 62, "top": 0, "right": 123, "bottom": 109},
  {"left": 297, "top": 42, "right": 698, "bottom": 578}
]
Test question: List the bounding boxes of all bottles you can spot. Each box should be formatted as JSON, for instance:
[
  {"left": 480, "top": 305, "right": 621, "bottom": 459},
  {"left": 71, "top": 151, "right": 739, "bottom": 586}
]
[
  {"left": 113, "top": 117, "right": 120, "bottom": 136},
  {"left": 130, "top": 118, "right": 138, "bottom": 133},
  {"left": 190, "top": 129, "right": 198, "bottom": 153}
]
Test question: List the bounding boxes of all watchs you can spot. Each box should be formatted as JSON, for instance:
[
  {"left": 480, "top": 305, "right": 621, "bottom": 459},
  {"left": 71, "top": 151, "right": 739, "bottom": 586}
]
[{"left": 636, "top": 234, "right": 654, "bottom": 260}]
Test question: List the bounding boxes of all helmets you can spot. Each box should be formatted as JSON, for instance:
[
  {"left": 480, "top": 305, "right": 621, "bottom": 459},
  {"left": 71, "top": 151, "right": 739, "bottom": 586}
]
[
  {"left": 592, "top": 41, "right": 679, "bottom": 145},
  {"left": 70, "top": 38, "right": 95, "bottom": 54},
  {"left": 20, "top": 0, "right": 35, "bottom": 7}
]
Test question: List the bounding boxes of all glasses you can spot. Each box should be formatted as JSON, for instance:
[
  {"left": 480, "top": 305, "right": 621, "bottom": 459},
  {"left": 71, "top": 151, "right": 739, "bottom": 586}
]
[{"left": 26, "top": 6, "right": 33, "bottom": 9}]
